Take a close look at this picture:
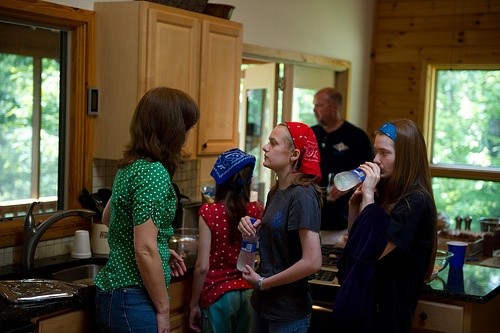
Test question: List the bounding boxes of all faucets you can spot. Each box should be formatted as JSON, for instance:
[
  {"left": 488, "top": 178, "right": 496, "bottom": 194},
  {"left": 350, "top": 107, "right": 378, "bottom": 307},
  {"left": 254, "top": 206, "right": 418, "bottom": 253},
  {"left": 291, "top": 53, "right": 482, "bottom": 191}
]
[{"left": 22, "top": 202, "right": 95, "bottom": 270}]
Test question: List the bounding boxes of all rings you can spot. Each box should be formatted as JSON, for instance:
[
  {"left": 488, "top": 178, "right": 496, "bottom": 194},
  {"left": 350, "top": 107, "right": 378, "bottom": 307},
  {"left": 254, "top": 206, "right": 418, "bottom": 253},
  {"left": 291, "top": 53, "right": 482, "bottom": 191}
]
[{"left": 170, "top": 263, "right": 174, "bottom": 265}]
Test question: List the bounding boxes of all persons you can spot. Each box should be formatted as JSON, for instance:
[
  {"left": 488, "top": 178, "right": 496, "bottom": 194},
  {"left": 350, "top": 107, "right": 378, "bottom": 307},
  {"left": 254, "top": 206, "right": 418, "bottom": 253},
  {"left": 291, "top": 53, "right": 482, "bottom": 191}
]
[
  {"left": 191, "top": 148, "right": 265, "bottom": 333},
  {"left": 238, "top": 122, "right": 322, "bottom": 333},
  {"left": 94, "top": 86, "right": 199, "bottom": 333},
  {"left": 337, "top": 119, "right": 438, "bottom": 333},
  {"left": 309, "top": 88, "right": 374, "bottom": 243}
]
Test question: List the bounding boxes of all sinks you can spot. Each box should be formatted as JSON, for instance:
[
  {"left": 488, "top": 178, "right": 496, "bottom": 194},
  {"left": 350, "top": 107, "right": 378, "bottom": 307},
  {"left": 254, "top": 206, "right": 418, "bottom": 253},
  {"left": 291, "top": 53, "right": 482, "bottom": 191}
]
[{"left": 42, "top": 256, "right": 110, "bottom": 289}]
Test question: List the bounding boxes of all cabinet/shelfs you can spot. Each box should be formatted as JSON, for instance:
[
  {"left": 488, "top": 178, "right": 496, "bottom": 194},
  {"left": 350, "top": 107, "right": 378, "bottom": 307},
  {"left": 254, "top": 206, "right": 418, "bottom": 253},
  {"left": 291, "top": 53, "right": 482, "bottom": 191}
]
[
  {"left": 409, "top": 294, "right": 500, "bottom": 333},
  {"left": 90, "top": 1, "right": 243, "bottom": 159}
]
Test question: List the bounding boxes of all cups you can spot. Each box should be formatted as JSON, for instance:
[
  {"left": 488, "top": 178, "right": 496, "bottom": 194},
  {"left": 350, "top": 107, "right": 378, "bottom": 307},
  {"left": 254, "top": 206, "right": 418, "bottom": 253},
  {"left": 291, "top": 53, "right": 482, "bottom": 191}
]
[
  {"left": 447, "top": 240, "right": 468, "bottom": 272},
  {"left": 480, "top": 217, "right": 500, "bottom": 232},
  {"left": 93, "top": 222, "right": 110, "bottom": 254},
  {"left": 72, "top": 230, "right": 91, "bottom": 258},
  {"left": 447, "top": 268, "right": 464, "bottom": 292}
]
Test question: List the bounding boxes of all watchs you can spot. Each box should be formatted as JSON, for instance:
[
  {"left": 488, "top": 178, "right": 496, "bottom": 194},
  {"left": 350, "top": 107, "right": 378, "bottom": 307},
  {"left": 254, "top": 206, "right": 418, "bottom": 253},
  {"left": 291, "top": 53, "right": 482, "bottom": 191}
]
[{"left": 256, "top": 277, "right": 266, "bottom": 291}]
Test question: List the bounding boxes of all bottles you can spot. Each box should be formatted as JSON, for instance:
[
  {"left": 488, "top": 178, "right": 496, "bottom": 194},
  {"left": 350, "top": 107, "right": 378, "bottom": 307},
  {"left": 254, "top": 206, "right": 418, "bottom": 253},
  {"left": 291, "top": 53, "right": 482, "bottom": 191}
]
[
  {"left": 236, "top": 217, "right": 257, "bottom": 275},
  {"left": 454, "top": 215, "right": 463, "bottom": 230},
  {"left": 484, "top": 232, "right": 493, "bottom": 258},
  {"left": 334, "top": 168, "right": 366, "bottom": 192},
  {"left": 464, "top": 214, "right": 472, "bottom": 230}
]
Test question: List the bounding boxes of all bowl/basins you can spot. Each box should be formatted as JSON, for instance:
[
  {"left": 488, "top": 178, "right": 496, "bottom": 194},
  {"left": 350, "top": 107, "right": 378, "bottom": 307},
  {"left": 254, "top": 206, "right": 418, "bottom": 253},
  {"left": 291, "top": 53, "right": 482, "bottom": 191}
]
[
  {"left": 432, "top": 250, "right": 454, "bottom": 274},
  {"left": 168, "top": 227, "right": 201, "bottom": 260}
]
